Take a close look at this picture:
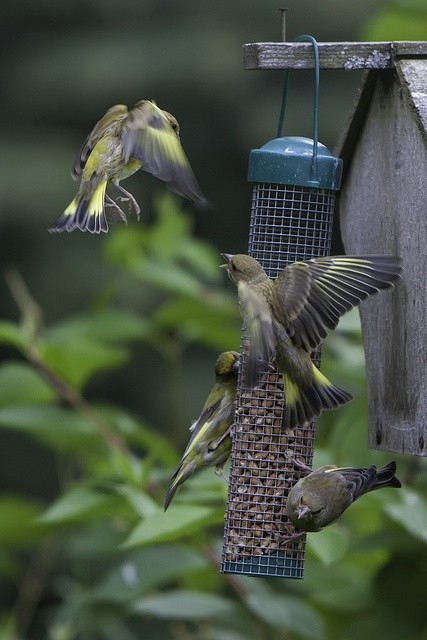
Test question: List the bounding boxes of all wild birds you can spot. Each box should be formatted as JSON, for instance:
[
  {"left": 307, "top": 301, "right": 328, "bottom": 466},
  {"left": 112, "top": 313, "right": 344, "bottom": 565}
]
[
  {"left": 48, "top": 100, "right": 208, "bottom": 234},
  {"left": 281, "top": 456, "right": 404, "bottom": 548},
  {"left": 164, "top": 352, "right": 241, "bottom": 514},
  {"left": 219, "top": 252, "right": 405, "bottom": 433}
]
